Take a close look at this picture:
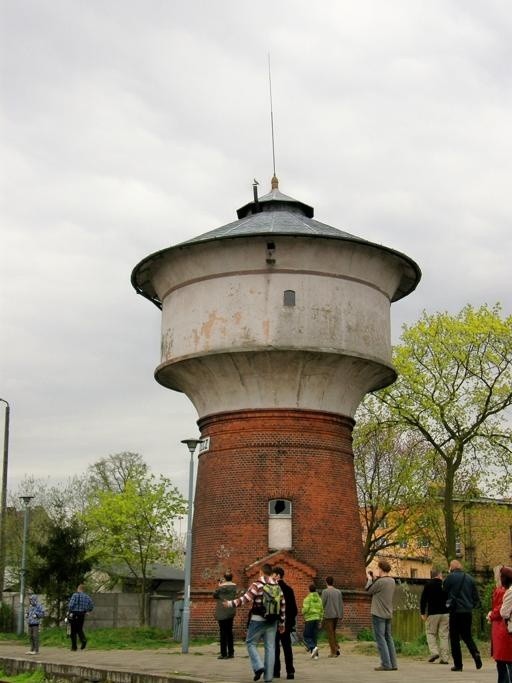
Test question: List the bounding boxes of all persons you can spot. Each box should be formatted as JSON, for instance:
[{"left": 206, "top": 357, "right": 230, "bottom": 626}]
[
  {"left": 66, "top": 608, "right": 73, "bottom": 638},
  {"left": 25, "top": 595, "right": 45, "bottom": 653},
  {"left": 365, "top": 560, "right": 400, "bottom": 670},
  {"left": 302, "top": 585, "right": 324, "bottom": 659},
  {"left": 213, "top": 571, "right": 237, "bottom": 659},
  {"left": 420, "top": 566, "right": 451, "bottom": 663},
  {"left": 69, "top": 584, "right": 93, "bottom": 651},
  {"left": 500, "top": 586, "right": 512, "bottom": 634},
  {"left": 443, "top": 560, "right": 481, "bottom": 671},
  {"left": 224, "top": 563, "right": 286, "bottom": 683},
  {"left": 272, "top": 567, "right": 299, "bottom": 677},
  {"left": 486, "top": 567, "right": 512, "bottom": 682},
  {"left": 320, "top": 576, "right": 343, "bottom": 658}
]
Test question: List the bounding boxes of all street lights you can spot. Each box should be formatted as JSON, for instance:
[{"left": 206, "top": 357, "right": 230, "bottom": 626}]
[
  {"left": 180, "top": 437, "right": 205, "bottom": 652},
  {"left": 16, "top": 494, "right": 32, "bottom": 640}
]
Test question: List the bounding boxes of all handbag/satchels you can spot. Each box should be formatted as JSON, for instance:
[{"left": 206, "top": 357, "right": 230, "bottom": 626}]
[{"left": 444, "top": 598, "right": 457, "bottom": 611}]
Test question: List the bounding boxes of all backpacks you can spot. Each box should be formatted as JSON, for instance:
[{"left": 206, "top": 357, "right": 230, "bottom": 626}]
[{"left": 254, "top": 581, "right": 281, "bottom": 620}]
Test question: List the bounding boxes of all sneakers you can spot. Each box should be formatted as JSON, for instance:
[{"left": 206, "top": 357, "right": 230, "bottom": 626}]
[
  {"left": 475, "top": 653, "right": 482, "bottom": 669},
  {"left": 25, "top": 651, "right": 40, "bottom": 655},
  {"left": 451, "top": 667, "right": 462, "bottom": 671},
  {"left": 254, "top": 669, "right": 265, "bottom": 681},
  {"left": 375, "top": 667, "right": 397, "bottom": 671},
  {"left": 311, "top": 646, "right": 340, "bottom": 660},
  {"left": 428, "top": 655, "right": 439, "bottom": 662}
]
